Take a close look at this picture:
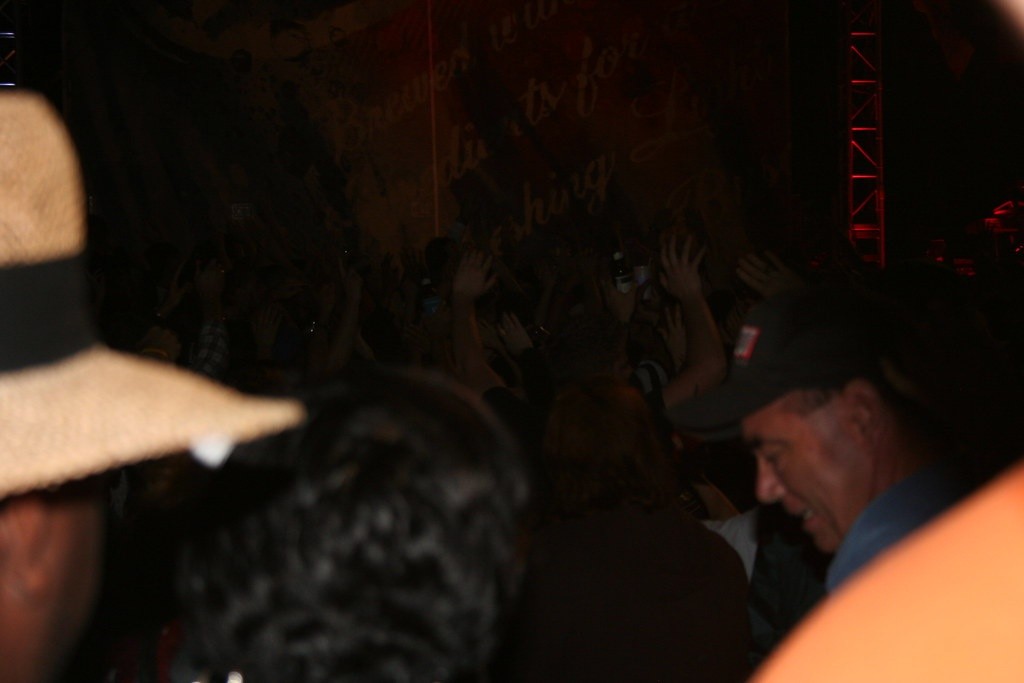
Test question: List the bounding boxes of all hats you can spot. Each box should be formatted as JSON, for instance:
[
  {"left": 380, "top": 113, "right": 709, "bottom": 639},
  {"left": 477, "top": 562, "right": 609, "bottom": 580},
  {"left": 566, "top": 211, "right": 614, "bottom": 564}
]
[
  {"left": 0, "top": 84, "right": 312, "bottom": 502},
  {"left": 665, "top": 289, "right": 893, "bottom": 440}
]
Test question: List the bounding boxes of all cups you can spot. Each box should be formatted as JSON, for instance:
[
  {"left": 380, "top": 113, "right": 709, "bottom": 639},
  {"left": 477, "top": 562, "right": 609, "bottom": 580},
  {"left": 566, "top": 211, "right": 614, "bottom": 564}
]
[{"left": 634, "top": 266, "right": 651, "bottom": 286}]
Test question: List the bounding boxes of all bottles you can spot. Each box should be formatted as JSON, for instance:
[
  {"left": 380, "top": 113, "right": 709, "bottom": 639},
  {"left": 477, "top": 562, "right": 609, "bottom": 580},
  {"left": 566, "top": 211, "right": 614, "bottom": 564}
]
[
  {"left": 614, "top": 253, "right": 631, "bottom": 295},
  {"left": 533, "top": 320, "right": 550, "bottom": 337}
]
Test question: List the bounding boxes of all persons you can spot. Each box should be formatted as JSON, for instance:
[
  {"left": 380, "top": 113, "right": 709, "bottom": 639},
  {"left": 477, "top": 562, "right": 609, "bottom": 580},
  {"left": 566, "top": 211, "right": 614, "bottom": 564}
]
[{"left": 0, "top": 87, "right": 1023, "bottom": 683}]
